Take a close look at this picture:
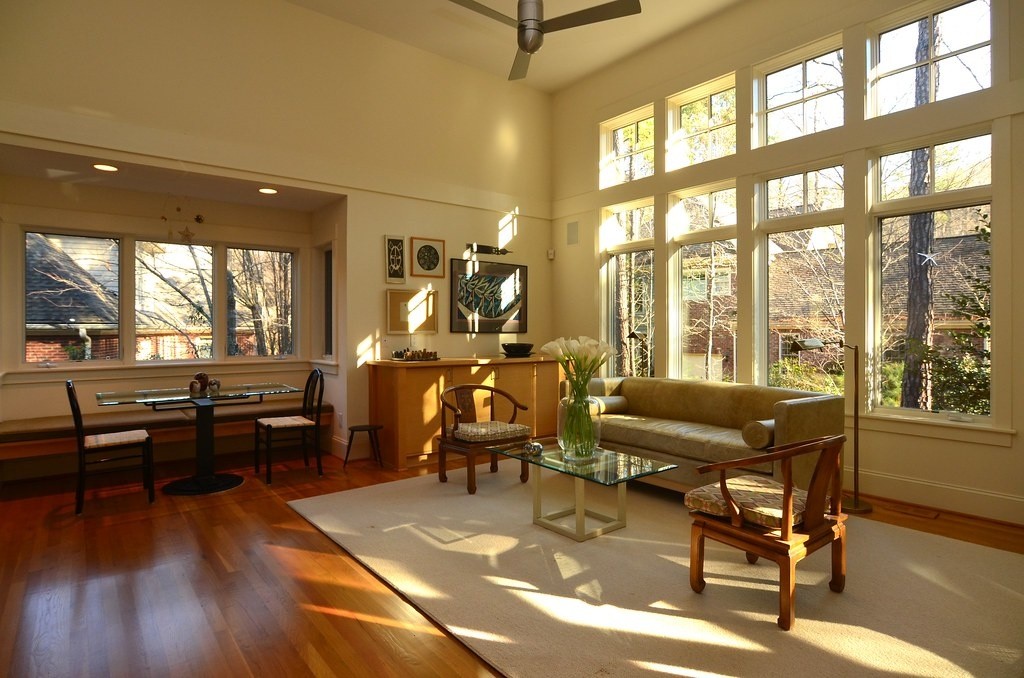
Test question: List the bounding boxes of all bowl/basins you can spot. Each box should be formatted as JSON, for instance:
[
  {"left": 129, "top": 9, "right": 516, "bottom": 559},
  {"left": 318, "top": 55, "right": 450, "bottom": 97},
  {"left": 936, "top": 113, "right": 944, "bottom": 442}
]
[{"left": 502, "top": 343, "right": 533, "bottom": 353}]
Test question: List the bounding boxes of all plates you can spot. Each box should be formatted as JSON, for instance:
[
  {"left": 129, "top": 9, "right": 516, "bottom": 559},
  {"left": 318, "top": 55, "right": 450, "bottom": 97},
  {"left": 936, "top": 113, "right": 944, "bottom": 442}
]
[{"left": 501, "top": 352, "right": 535, "bottom": 357}]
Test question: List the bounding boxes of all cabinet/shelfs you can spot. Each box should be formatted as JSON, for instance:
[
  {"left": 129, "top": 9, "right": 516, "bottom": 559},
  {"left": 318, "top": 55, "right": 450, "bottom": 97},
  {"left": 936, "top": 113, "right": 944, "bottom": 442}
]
[{"left": 365, "top": 354, "right": 576, "bottom": 472}]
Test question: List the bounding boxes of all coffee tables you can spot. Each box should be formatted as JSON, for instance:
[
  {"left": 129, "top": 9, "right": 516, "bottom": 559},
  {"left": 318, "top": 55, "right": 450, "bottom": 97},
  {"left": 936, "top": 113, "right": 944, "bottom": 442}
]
[{"left": 486, "top": 436, "right": 679, "bottom": 543}]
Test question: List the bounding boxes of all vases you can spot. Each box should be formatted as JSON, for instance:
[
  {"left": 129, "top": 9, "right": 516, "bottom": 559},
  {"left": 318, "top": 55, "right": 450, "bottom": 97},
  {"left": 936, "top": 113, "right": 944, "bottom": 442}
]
[{"left": 557, "top": 371, "right": 602, "bottom": 461}]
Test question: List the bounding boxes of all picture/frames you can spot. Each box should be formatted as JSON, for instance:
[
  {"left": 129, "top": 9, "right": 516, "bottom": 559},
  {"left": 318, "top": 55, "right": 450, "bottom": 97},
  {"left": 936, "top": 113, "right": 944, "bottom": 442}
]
[
  {"left": 450, "top": 258, "right": 528, "bottom": 334},
  {"left": 384, "top": 234, "right": 406, "bottom": 284},
  {"left": 385, "top": 288, "right": 439, "bottom": 335},
  {"left": 410, "top": 237, "right": 446, "bottom": 278}
]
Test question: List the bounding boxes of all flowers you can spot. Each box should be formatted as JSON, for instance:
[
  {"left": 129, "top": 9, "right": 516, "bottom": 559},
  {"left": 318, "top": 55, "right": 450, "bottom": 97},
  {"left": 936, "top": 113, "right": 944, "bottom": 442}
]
[{"left": 539, "top": 335, "right": 618, "bottom": 457}]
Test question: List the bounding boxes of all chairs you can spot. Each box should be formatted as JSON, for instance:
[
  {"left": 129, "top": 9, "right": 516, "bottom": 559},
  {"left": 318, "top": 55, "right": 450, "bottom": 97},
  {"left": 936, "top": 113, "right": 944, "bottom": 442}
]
[
  {"left": 254, "top": 366, "right": 324, "bottom": 484},
  {"left": 436, "top": 383, "right": 532, "bottom": 494},
  {"left": 683, "top": 434, "right": 848, "bottom": 630},
  {"left": 65, "top": 379, "right": 153, "bottom": 516}
]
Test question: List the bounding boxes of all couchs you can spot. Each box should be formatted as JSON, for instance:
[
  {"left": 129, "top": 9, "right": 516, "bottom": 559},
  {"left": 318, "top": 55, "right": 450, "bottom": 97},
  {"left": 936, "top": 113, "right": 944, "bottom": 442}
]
[{"left": 586, "top": 375, "right": 845, "bottom": 493}]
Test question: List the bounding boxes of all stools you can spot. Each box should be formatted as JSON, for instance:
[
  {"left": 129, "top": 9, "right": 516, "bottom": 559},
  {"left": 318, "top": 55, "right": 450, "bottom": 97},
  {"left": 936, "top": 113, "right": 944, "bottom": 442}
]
[{"left": 343, "top": 424, "right": 383, "bottom": 468}]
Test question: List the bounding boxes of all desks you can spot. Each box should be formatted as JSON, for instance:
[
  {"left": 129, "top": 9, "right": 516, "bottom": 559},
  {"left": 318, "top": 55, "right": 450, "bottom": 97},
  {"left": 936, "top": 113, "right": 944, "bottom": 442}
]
[{"left": 96, "top": 382, "right": 304, "bottom": 496}]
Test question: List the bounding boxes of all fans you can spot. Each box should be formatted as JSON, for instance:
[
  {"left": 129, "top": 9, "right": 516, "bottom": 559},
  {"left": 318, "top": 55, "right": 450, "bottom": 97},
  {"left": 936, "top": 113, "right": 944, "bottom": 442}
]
[{"left": 451, "top": 0, "right": 642, "bottom": 80}]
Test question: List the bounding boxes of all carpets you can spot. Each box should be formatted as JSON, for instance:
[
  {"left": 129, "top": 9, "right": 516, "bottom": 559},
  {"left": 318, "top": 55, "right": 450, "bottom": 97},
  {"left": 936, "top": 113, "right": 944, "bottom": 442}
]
[{"left": 283, "top": 458, "right": 1024, "bottom": 678}]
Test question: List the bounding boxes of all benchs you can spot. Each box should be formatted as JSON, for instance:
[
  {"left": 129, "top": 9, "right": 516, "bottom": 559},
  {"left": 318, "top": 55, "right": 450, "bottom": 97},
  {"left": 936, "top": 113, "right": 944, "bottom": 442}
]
[{"left": 0, "top": 397, "right": 334, "bottom": 461}]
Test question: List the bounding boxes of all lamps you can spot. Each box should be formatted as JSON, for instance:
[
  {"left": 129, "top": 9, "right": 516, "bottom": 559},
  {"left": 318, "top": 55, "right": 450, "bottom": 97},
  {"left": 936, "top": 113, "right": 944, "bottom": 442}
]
[{"left": 789, "top": 337, "right": 873, "bottom": 514}]
